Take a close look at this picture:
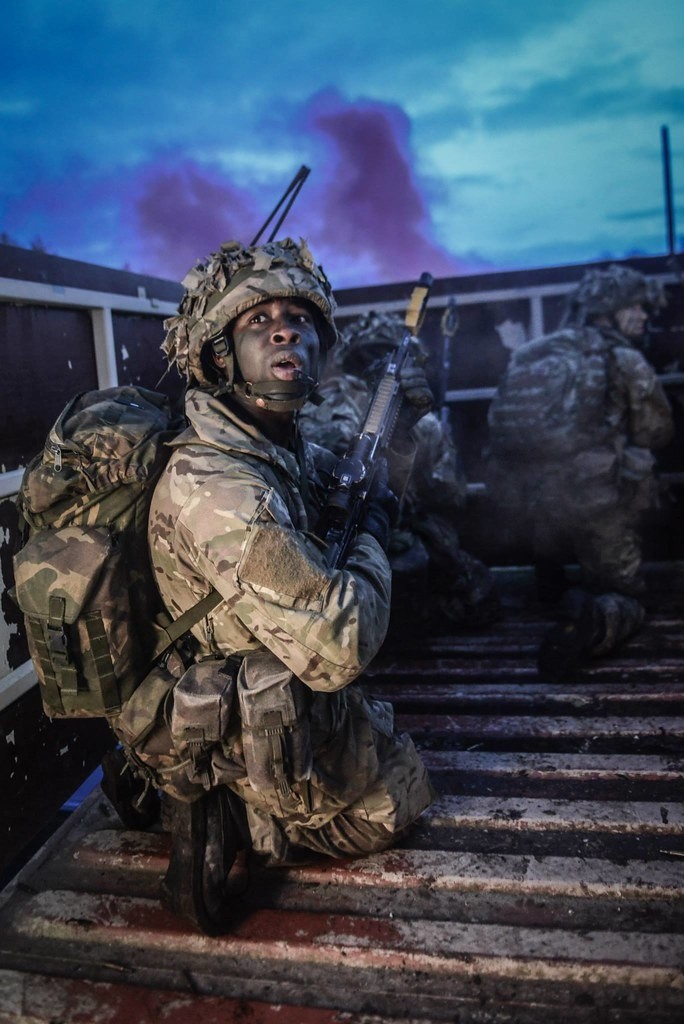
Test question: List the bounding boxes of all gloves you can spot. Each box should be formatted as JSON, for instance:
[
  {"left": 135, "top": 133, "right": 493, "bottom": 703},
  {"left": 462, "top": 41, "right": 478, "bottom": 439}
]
[
  {"left": 360, "top": 480, "right": 400, "bottom": 548},
  {"left": 362, "top": 357, "right": 434, "bottom": 437}
]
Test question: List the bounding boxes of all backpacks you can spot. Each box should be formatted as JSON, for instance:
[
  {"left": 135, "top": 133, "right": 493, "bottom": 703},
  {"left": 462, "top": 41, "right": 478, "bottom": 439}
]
[
  {"left": 12, "top": 386, "right": 308, "bottom": 719},
  {"left": 486, "top": 327, "right": 629, "bottom": 460}
]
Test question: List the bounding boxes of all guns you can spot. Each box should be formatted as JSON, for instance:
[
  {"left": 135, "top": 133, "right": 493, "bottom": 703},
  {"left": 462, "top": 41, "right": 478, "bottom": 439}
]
[
  {"left": 317, "top": 270, "right": 435, "bottom": 580},
  {"left": 440, "top": 293, "right": 462, "bottom": 425}
]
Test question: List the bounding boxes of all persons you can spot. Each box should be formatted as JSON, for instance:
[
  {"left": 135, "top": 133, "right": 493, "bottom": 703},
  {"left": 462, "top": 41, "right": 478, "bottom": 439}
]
[
  {"left": 327, "top": 309, "right": 478, "bottom": 664},
  {"left": 479, "top": 268, "right": 667, "bottom": 667},
  {"left": 109, "top": 242, "right": 439, "bottom": 933}
]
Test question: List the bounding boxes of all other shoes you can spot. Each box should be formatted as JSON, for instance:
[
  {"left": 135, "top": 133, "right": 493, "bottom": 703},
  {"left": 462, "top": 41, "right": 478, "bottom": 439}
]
[
  {"left": 100, "top": 746, "right": 163, "bottom": 832},
  {"left": 537, "top": 587, "right": 594, "bottom": 684},
  {"left": 159, "top": 784, "right": 250, "bottom": 938},
  {"left": 536, "top": 560, "right": 571, "bottom": 600}
]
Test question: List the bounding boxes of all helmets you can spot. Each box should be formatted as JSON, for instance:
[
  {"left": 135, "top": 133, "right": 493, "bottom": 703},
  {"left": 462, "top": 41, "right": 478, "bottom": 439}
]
[
  {"left": 160, "top": 236, "right": 338, "bottom": 412},
  {"left": 566, "top": 262, "right": 651, "bottom": 327},
  {"left": 336, "top": 308, "right": 428, "bottom": 369}
]
